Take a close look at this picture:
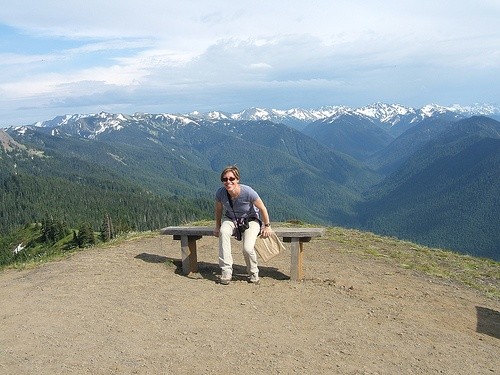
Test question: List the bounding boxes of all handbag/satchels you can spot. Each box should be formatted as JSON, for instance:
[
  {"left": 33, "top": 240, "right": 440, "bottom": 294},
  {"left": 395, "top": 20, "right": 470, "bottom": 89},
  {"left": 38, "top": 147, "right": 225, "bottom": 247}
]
[{"left": 254, "top": 231, "right": 285, "bottom": 263}]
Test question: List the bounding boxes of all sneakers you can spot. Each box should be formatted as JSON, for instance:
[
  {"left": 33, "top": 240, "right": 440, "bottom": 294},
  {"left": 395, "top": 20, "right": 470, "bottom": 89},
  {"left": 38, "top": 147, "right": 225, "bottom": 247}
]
[
  {"left": 220, "top": 269, "right": 233, "bottom": 285},
  {"left": 250, "top": 273, "right": 258, "bottom": 282}
]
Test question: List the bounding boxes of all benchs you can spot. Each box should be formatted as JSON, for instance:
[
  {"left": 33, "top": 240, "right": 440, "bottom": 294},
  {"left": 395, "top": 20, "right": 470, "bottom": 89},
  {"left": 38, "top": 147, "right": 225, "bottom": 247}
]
[{"left": 159, "top": 225, "right": 326, "bottom": 282}]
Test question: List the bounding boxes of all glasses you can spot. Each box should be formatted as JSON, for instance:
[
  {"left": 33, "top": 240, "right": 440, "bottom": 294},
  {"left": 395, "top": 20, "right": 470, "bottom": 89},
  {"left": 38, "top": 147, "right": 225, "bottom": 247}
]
[{"left": 222, "top": 177, "right": 238, "bottom": 181}]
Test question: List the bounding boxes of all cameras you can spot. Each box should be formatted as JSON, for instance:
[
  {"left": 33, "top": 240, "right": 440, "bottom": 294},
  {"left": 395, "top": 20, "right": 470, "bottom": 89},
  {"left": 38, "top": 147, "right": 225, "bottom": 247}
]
[{"left": 238, "top": 219, "right": 249, "bottom": 233}]
